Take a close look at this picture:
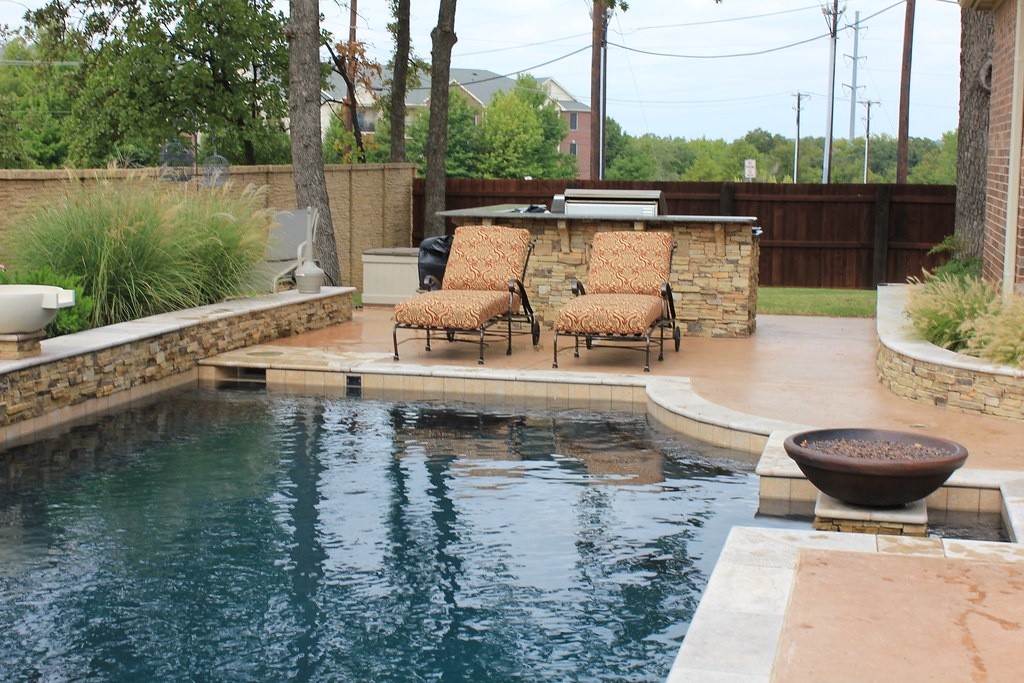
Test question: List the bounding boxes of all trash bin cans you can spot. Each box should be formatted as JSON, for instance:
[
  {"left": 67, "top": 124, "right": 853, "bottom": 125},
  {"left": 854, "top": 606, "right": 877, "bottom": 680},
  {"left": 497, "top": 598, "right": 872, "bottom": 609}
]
[{"left": 418, "top": 234, "right": 454, "bottom": 293}]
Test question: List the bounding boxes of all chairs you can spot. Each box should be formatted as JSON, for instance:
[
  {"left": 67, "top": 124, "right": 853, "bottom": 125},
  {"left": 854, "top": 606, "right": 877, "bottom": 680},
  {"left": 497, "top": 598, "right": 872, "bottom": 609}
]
[
  {"left": 255, "top": 207, "right": 334, "bottom": 293},
  {"left": 552, "top": 231, "right": 681, "bottom": 372},
  {"left": 392, "top": 226, "right": 541, "bottom": 365}
]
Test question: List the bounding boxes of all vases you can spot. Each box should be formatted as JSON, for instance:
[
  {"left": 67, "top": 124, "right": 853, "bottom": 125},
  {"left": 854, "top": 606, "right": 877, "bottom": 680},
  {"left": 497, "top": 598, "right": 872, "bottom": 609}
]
[
  {"left": 0, "top": 283, "right": 75, "bottom": 335},
  {"left": 783, "top": 427, "right": 968, "bottom": 508}
]
